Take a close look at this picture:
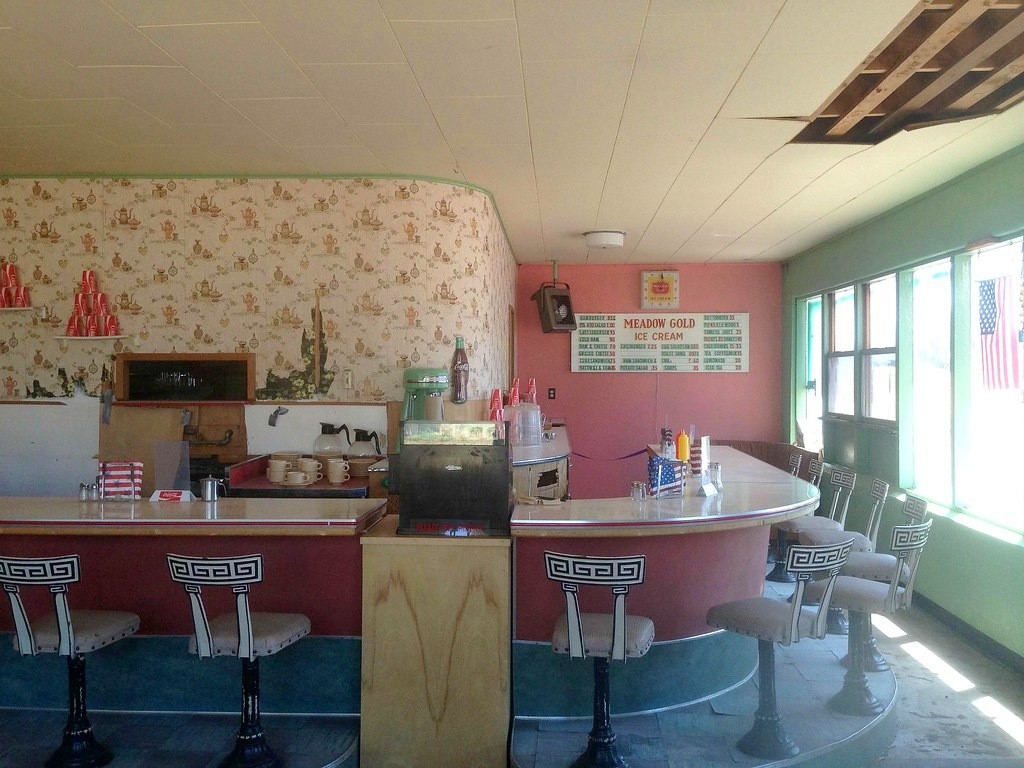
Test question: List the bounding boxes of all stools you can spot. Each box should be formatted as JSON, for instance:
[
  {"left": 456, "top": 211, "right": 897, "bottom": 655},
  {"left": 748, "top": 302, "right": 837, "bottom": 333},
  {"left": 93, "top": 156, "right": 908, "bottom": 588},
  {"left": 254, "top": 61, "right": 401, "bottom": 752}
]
[
  {"left": 801, "top": 518, "right": 933, "bottom": 717},
  {"left": 706, "top": 538, "right": 854, "bottom": 759},
  {"left": 767, "top": 451, "right": 802, "bottom": 563},
  {"left": 544, "top": 550, "right": 655, "bottom": 768},
  {"left": 800, "top": 480, "right": 890, "bottom": 633},
  {"left": 0, "top": 554, "right": 141, "bottom": 768},
  {"left": 165, "top": 552, "right": 311, "bottom": 768},
  {"left": 766, "top": 457, "right": 824, "bottom": 582},
  {"left": 777, "top": 468, "right": 857, "bottom": 604},
  {"left": 811, "top": 488, "right": 929, "bottom": 671}
]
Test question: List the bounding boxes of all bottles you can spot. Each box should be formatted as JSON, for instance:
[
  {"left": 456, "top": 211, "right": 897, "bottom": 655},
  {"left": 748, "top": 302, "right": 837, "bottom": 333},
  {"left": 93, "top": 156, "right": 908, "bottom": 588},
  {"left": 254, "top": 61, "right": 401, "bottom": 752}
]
[
  {"left": 630, "top": 481, "right": 647, "bottom": 501},
  {"left": 710, "top": 463, "right": 721, "bottom": 484},
  {"left": 80, "top": 483, "right": 89, "bottom": 501},
  {"left": 452, "top": 338, "right": 469, "bottom": 404},
  {"left": 676, "top": 429, "right": 682, "bottom": 459},
  {"left": 679, "top": 431, "right": 688, "bottom": 461},
  {"left": 90, "top": 483, "right": 98, "bottom": 501},
  {"left": 663, "top": 442, "right": 675, "bottom": 455}
]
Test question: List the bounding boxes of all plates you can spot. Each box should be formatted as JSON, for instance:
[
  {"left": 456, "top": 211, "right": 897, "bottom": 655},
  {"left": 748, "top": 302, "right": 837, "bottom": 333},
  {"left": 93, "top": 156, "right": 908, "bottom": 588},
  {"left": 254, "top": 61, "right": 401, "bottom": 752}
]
[{"left": 281, "top": 483, "right": 310, "bottom": 487}]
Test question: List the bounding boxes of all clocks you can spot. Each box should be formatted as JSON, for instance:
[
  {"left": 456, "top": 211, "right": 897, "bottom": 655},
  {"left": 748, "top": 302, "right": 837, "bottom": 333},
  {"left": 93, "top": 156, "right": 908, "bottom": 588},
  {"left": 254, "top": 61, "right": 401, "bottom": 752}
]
[{"left": 639, "top": 270, "right": 681, "bottom": 311}]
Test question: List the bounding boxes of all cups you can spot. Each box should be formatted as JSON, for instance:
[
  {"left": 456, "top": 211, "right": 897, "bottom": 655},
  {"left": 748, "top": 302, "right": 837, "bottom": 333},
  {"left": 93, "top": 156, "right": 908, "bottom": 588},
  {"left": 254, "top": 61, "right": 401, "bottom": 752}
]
[
  {"left": 522, "top": 378, "right": 537, "bottom": 404},
  {"left": 74, "top": 293, "right": 89, "bottom": 316},
  {"left": 67, "top": 316, "right": 100, "bottom": 336},
  {"left": 104, "top": 316, "right": 119, "bottom": 336},
  {"left": 200, "top": 475, "right": 226, "bottom": 502},
  {"left": 92, "top": 293, "right": 109, "bottom": 316},
  {"left": 490, "top": 388, "right": 504, "bottom": 422},
  {"left": 328, "top": 459, "right": 350, "bottom": 485},
  {"left": 297, "top": 458, "right": 323, "bottom": 484},
  {"left": 287, "top": 472, "right": 309, "bottom": 483},
  {"left": 508, "top": 377, "right": 520, "bottom": 407},
  {"left": 82, "top": 271, "right": 96, "bottom": 294},
  {"left": 0, "top": 264, "right": 30, "bottom": 308},
  {"left": 267, "top": 460, "right": 292, "bottom": 483}
]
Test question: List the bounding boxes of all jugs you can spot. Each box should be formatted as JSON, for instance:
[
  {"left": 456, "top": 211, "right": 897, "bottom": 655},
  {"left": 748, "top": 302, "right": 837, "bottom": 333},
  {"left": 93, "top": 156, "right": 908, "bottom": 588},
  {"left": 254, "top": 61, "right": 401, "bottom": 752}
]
[
  {"left": 313, "top": 422, "right": 352, "bottom": 476},
  {"left": 347, "top": 429, "right": 381, "bottom": 477}
]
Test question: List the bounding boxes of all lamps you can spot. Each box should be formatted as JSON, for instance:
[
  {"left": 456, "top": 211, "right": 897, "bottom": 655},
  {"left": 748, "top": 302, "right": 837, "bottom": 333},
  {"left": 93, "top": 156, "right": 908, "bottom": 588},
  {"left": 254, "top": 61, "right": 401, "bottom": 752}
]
[{"left": 583, "top": 230, "right": 627, "bottom": 249}]
